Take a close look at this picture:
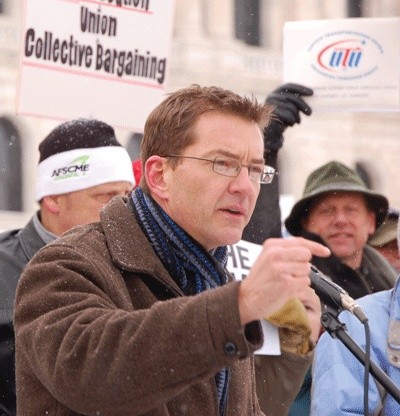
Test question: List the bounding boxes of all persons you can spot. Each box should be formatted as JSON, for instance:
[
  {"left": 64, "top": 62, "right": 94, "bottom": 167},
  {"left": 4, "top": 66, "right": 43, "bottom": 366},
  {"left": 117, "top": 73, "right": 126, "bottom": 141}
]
[
  {"left": 309, "top": 274, "right": 400, "bottom": 416},
  {"left": 242, "top": 80, "right": 400, "bottom": 416},
  {"left": 13, "top": 85, "right": 330, "bottom": 416},
  {"left": 366, "top": 211, "right": 400, "bottom": 273},
  {"left": 0, "top": 119, "right": 136, "bottom": 416}
]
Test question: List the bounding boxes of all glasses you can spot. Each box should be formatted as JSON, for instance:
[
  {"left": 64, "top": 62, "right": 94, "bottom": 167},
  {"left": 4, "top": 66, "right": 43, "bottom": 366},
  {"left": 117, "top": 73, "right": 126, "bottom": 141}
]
[{"left": 159, "top": 154, "right": 278, "bottom": 185}]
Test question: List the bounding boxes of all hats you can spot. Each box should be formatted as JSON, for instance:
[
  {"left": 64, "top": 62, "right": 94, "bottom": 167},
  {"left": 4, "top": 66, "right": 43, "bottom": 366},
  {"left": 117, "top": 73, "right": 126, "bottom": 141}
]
[
  {"left": 284, "top": 161, "right": 389, "bottom": 237},
  {"left": 368, "top": 219, "right": 398, "bottom": 247},
  {"left": 36, "top": 119, "right": 135, "bottom": 202}
]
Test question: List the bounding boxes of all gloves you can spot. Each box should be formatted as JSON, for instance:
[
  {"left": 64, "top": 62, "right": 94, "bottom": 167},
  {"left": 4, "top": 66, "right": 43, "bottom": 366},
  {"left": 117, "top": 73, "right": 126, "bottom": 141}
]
[{"left": 264, "top": 84, "right": 314, "bottom": 153}]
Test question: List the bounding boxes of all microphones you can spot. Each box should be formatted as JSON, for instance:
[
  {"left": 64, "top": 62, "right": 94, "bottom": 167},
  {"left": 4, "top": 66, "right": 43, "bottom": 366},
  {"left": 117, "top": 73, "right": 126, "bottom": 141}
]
[{"left": 309, "top": 264, "right": 367, "bottom": 326}]
